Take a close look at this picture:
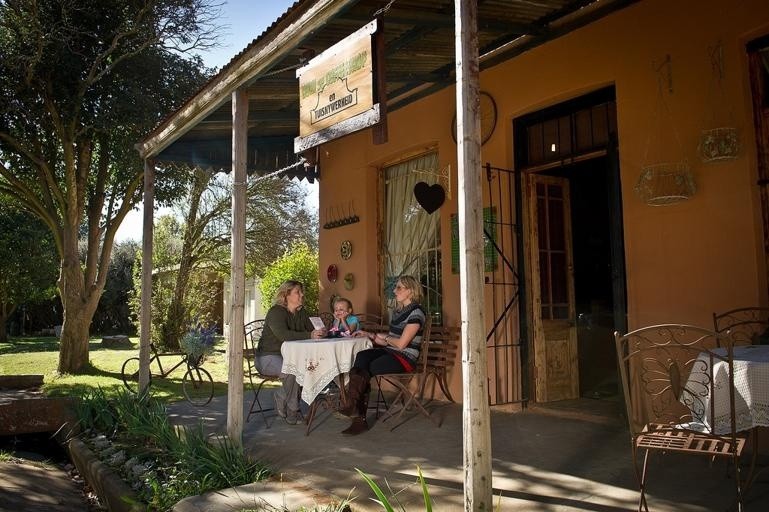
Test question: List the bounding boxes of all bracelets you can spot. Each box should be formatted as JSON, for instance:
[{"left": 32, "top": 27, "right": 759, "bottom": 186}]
[
  {"left": 385, "top": 335, "right": 391, "bottom": 342},
  {"left": 372, "top": 333, "right": 377, "bottom": 340}
]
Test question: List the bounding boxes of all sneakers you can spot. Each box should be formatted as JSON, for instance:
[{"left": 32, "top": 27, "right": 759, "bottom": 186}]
[{"left": 274, "top": 391, "right": 297, "bottom": 425}]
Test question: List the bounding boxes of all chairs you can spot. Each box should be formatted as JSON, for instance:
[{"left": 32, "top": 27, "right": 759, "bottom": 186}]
[
  {"left": 242, "top": 312, "right": 439, "bottom": 428},
  {"left": 713, "top": 307, "right": 769, "bottom": 483},
  {"left": 614, "top": 324, "right": 758, "bottom": 512}
]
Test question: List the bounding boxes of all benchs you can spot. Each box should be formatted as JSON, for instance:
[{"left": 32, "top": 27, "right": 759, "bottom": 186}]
[{"left": 362, "top": 325, "right": 461, "bottom": 403}]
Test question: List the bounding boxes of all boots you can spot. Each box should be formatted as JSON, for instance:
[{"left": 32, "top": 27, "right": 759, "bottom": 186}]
[{"left": 338, "top": 368, "right": 371, "bottom": 435}]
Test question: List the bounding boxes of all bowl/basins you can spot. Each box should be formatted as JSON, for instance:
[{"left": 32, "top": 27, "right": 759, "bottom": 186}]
[{"left": 327, "top": 331, "right": 340, "bottom": 338}]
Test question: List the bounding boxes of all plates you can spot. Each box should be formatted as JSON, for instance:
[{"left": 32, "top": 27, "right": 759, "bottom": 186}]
[
  {"left": 329, "top": 292, "right": 341, "bottom": 314},
  {"left": 340, "top": 240, "right": 352, "bottom": 260},
  {"left": 342, "top": 273, "right": 354, "bottom": 291},
  {"left": 327, "top": 264, "right": 337, "bottom": 283}
]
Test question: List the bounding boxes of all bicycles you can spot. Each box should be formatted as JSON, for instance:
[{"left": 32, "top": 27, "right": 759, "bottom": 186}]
[{"left": 122, "top": 341, "right": 216, "bottom": 407}]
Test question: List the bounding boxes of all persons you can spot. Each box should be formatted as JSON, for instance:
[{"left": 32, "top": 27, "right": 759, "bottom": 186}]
[
  {"left": 254, "top": 280, "right": 326, "bottom": 425},
  {"left": 332, "top": 298, "right": 362, "bottom": 336},
  {"left": 334, "top": 275, "right": 426, "bottom": 436}
]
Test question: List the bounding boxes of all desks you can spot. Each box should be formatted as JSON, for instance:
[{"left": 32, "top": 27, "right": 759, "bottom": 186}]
[
  {"left": 280, "top": 338, "right": 374, "bottom": 437},
  {"left": 679, "top": 345, "right": 769, "bottom": 512}
]
